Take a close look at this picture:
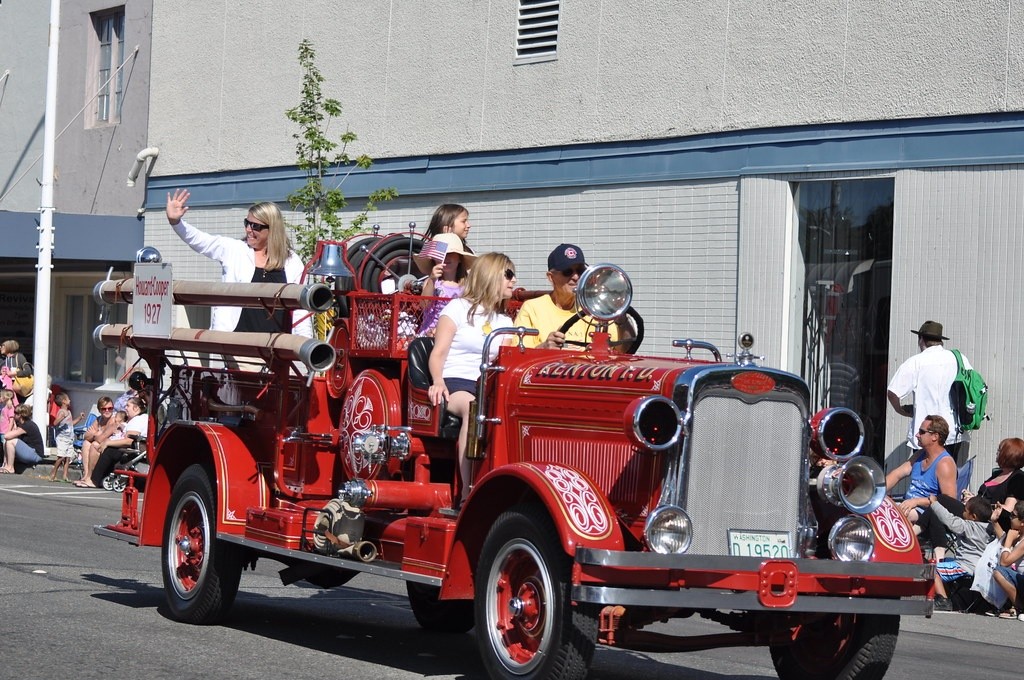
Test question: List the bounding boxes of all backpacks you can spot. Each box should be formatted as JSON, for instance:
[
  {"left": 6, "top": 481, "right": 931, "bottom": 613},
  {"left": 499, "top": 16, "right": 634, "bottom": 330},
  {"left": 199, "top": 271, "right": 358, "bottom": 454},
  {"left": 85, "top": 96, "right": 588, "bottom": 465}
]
[{"left": 951, "top": 349, "right": 990, "bottom": 442}]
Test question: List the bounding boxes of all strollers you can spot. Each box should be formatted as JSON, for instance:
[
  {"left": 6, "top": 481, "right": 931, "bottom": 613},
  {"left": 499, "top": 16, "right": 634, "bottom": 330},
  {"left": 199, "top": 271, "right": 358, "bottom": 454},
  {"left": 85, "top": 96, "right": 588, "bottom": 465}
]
[{"left": 102, "top": 434, "right": 148, "bottom": 493}]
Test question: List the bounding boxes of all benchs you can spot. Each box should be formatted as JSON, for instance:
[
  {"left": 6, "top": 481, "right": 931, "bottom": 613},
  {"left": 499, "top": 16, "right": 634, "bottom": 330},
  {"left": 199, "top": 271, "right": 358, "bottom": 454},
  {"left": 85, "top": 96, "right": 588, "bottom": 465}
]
[{"left": 404, "top": 337, "right": 464, "bottom": 436}]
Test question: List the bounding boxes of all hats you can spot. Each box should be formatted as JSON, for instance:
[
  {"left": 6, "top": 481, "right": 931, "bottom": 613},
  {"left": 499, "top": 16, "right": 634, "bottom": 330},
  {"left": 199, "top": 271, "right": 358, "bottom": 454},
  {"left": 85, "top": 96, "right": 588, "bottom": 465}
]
[
  {"left": 413, "top": 233, "right": 478, "bottom": 275},
  {"left": 548, "top": 243, "right": 590, "bottom": 271},
  {"left": 911, "top": 321, "right": 950, "bottom": 341}
]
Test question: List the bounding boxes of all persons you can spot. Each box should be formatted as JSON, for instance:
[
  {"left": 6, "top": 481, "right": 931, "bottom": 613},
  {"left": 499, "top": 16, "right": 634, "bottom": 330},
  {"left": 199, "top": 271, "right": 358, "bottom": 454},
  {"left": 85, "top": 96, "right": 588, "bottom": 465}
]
[
  {"left": 888, "top": 320, "right": 976, "bottom": 468},
  {"left": 0, "top": 339, "right": 164, "bottom": 488},
  {"left": 411, "top": 204, "right": 479, "bottom": 337},
  {"left": 427, "top": 252, "right": 518, "bottom": 509},
  {"left": 165, "top": 189, "right": 313, "bottom": 376},
  {"left": 513, "top": 243, "right": 635, "bottom": 352},
  {"left": 887, "top": 415, "right": 1024, "bottom": 620},
  {"left": 826, "top": 341, "right": 863, "bottom": 415}
]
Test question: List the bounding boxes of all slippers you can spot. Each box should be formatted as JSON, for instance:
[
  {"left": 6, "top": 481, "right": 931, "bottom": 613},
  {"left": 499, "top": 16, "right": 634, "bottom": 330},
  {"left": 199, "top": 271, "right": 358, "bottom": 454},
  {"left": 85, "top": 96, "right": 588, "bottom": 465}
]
[
  {"left": 60, "top": 478, "right": 71, "bottom": 483},
  {"left": 72, "top": 480, "right": 84, "bottom": 485},
  {"left": 75, "top": 481, "right": 92, "bottom": 488},
  {"left": 0, "top": 466, "right": 15, "bottom": 474}
]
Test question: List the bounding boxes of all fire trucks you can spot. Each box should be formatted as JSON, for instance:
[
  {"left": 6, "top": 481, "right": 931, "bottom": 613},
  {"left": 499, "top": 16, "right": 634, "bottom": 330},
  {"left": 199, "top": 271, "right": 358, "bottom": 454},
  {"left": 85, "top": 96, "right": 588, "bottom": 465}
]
[{"left": 92, "top": 223, "right": 935, "bottom": 680}]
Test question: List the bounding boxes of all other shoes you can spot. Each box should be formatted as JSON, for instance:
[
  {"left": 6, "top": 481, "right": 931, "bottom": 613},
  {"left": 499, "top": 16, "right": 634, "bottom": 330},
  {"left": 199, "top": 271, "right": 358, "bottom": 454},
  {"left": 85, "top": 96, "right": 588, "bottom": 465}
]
[
  {"left": 985, "top": 607, "right": 1006, "bottom": 617},
  {"left": 1018, "top": 614, "right": 1024, "bottom": 621},
  {"left": 48, "top": 475, "right": 60, "bottom": 482},
  {"left": 456, "top": 496, "right": 467, "bottom": 510},
  {"left": 999, "top": 606, "right": 1017, "bottom": 619},
  {"left": 934, "top": 594, "right": 953, "bottom": 610}
]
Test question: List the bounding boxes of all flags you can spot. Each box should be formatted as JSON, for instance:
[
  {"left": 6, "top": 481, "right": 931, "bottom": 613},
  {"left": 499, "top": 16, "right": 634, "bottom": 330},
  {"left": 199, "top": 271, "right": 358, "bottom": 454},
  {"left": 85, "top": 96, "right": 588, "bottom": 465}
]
[{"left": 420, "top": 241, "right": 448, "bottom": 263}]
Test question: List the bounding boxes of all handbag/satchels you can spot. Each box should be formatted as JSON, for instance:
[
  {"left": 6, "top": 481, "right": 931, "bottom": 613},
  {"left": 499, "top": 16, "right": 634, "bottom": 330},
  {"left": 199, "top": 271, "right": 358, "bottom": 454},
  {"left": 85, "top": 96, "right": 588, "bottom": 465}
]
[
  {"left": 969, "top": 531, "right": 1009, "bottom": 609},
  {"left": 13, "top": 367, "right": 34, "bottom": 396}
]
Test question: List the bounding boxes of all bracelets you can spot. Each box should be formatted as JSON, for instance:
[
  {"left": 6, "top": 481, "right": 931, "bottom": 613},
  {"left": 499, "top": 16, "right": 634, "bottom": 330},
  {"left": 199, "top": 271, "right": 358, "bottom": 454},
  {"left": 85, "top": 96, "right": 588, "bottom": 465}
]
[
  {"left": 615, "top": 316, "right": 628, "bottom": 328},
  {"left": 1001, "top": 547, "right": 1011, "bottom": 554}
]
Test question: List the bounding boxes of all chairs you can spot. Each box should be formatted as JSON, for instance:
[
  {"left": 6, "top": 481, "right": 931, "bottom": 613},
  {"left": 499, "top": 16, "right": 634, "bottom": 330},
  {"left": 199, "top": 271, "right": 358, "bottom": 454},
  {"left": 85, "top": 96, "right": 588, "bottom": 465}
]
[
  {"left": 887, "top": 455, "right": 998, "bottom": 617},
  {"left": 73, "top": 403, "right": 101, "bottom": 450}
]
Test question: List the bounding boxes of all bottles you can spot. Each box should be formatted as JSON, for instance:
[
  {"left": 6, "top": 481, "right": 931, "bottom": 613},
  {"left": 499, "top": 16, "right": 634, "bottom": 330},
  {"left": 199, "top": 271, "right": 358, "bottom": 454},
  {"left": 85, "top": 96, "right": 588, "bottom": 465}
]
[{"left": 967, "top": 403, "right": 976, "bottom": 413}]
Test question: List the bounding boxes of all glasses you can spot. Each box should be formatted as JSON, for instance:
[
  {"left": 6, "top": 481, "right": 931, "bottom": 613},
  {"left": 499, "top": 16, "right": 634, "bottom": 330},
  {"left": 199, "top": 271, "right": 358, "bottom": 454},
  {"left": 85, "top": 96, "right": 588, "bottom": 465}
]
[
  {"left": 553, "top": 268, "right": 586, "bottom": 278},
  {"left": 505, "top": 269, "right": 515, "bottom": 280},
  {"left": 243, "top": 218, "right": 269, "bottom": 231},
  {"left": 1010, "top": 511, "right": 1021, "bottom": 519},
  {"left": 100, "top": 407, "right": 114, "bottom": 412},
  {"left": 919, "top": 429, "right": 937, "bottom": 435}
]
